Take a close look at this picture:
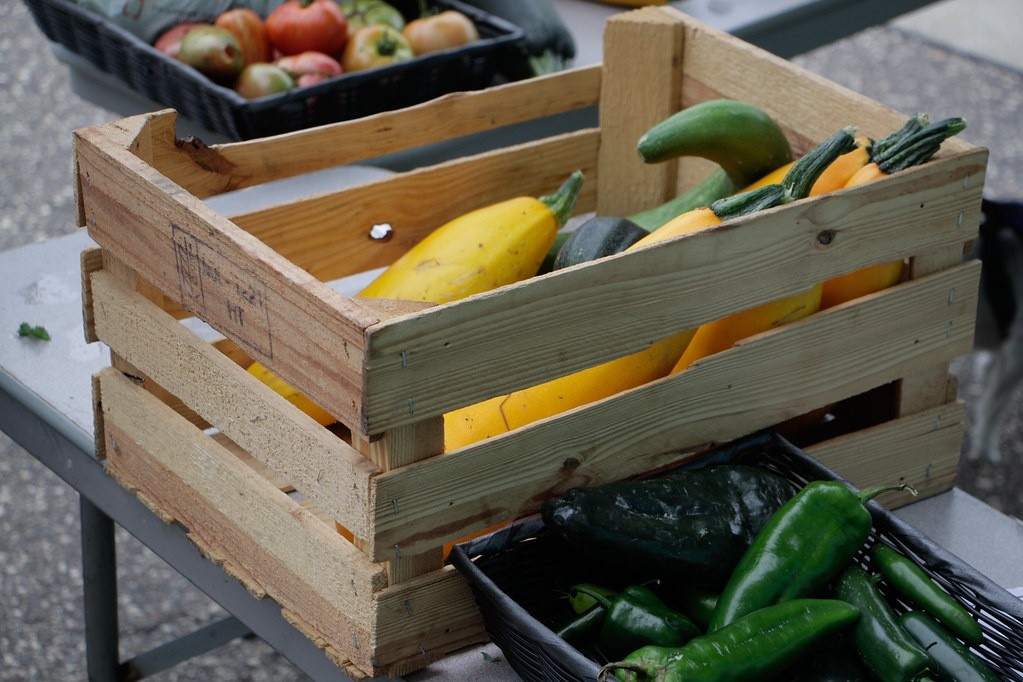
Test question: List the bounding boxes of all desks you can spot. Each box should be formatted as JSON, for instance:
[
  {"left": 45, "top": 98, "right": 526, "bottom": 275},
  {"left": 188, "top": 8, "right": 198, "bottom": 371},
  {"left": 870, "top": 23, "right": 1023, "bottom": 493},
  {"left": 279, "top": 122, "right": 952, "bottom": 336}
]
[{"left": 0, "top": 165, "right": 1023, "bottom": 682}]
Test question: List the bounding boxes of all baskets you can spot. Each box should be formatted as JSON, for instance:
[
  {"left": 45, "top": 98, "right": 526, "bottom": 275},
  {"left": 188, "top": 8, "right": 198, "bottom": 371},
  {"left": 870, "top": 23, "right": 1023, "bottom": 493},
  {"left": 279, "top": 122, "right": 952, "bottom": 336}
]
[
  {"left": 24, "top": 1, "right": 537, "bottom": 143},
  {"left": 445, "top": 431, "right": 1023, "bottom": 682}
]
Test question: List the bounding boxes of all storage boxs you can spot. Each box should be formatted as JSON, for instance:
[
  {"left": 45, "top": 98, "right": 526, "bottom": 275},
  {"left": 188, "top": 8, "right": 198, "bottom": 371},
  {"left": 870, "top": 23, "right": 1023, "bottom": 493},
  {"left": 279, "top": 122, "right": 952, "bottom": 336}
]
[
  {"left": 446, "top": 430, "right": 1023, "bottom": 682},
  {"left": 73, "top": 6, "right": 991, "bottom": 682}
]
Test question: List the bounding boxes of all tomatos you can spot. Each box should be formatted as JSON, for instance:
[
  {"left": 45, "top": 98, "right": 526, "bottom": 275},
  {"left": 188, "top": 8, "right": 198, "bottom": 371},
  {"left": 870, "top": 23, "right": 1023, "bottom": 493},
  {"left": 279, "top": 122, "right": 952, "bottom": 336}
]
[{"left": 149, "top": 0, "right": 481, "bottom": 101}]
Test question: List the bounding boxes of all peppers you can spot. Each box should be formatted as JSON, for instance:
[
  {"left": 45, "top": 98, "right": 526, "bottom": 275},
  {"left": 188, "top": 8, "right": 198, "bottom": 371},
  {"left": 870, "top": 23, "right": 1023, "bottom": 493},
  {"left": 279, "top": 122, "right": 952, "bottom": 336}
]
[{"left": 560, "top": 478, "right": 1001, "bottom": 682}]
